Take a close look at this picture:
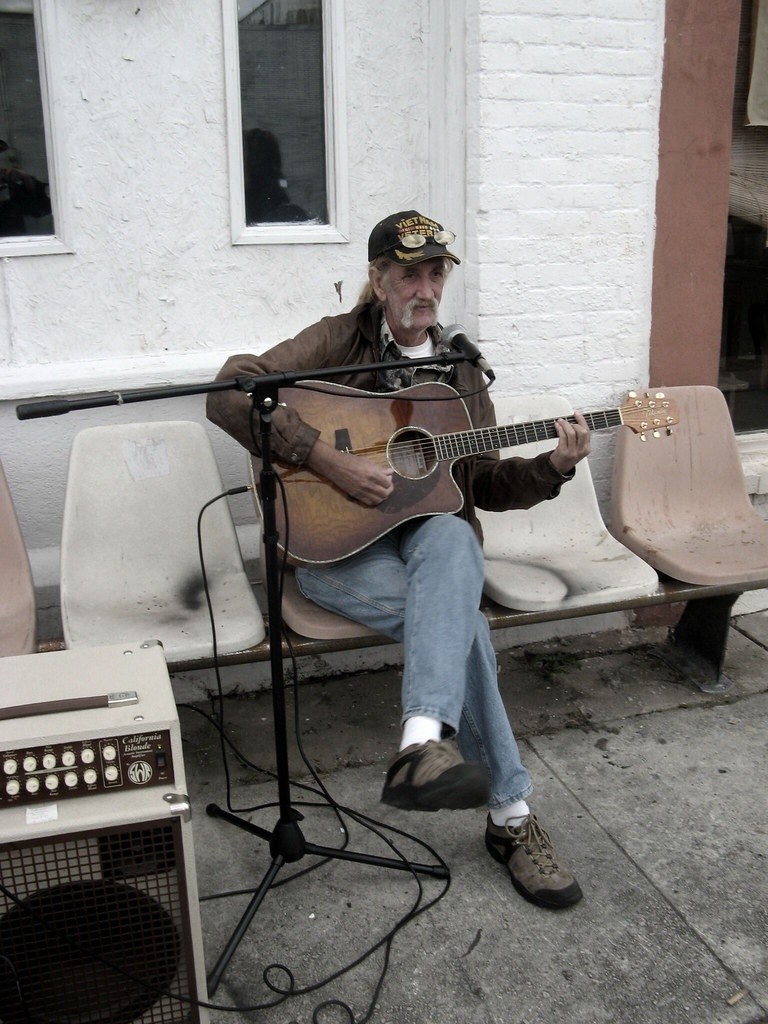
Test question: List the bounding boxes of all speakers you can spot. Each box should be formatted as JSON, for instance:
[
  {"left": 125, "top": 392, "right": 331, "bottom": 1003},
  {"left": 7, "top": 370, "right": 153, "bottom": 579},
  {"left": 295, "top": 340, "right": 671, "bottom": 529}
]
[{"left": 0, "top": 639, "right": 211, "bottom": 1024}]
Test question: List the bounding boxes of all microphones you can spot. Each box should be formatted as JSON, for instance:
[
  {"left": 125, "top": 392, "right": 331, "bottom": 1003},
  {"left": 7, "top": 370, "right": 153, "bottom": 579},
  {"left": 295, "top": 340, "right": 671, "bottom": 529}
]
[{"left": 441, "top": 323, "right": 496, "bottom": 381}]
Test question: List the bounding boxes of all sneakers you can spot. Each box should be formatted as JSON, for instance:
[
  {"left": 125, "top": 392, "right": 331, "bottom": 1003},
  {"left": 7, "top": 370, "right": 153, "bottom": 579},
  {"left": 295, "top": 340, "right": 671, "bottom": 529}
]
[
  {"left": 484, "top": 812, "right": 583, "bottom": 909},
  {"left": 380, "top": 739, "right": 491, "bottom": 812}
]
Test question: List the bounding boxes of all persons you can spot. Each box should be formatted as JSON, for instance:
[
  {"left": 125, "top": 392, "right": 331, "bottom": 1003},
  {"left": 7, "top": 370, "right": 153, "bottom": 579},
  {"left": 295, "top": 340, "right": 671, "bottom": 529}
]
[
  {"left": 243, "top": 128, "right": 308, "bottom": 222},
  {"left": 0, "top": 139, "right": 52, "bottom": 237},
  {"left": 205, "top": 210, "right": 592, "bottom": 910}
]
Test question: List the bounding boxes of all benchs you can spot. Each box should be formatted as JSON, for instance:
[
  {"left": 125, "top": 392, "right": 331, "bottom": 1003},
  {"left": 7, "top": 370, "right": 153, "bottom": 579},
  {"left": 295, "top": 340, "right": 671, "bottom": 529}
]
[{"left": 1, "top": 387, "right": 767, "bottom": 691}]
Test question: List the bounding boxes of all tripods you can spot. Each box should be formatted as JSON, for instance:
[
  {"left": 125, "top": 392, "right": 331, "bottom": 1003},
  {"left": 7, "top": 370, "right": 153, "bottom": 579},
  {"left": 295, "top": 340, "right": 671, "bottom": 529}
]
[{"left": 16, "top": 350, "right": 466, "bottom": 998}]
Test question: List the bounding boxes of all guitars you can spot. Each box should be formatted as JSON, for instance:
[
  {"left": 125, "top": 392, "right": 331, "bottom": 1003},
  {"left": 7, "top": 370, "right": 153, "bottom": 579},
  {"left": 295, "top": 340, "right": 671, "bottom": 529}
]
[{"left": 246, "top": 379, "right": 682, "bottom": 572}]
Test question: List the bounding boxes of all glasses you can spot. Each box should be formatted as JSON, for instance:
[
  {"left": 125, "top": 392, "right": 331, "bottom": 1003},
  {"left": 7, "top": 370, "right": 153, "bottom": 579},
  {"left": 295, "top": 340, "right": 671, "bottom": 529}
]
[{"left": 376, "top": 230, "right": 457, "bottom": 260}]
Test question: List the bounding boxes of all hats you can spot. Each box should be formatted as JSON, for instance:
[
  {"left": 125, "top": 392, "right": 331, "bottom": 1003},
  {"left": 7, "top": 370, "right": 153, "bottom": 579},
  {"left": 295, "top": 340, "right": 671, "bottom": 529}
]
[{"left": 367, "top": 210, "right": 461, "bottom": 267}]
